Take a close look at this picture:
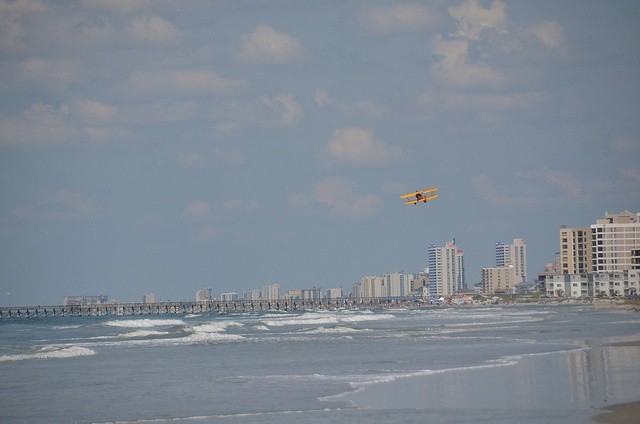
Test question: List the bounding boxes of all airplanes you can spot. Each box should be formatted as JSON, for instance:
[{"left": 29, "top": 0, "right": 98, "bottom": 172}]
[{"left": 399, "top": 188, "right": 439, "bottom": 205}]
